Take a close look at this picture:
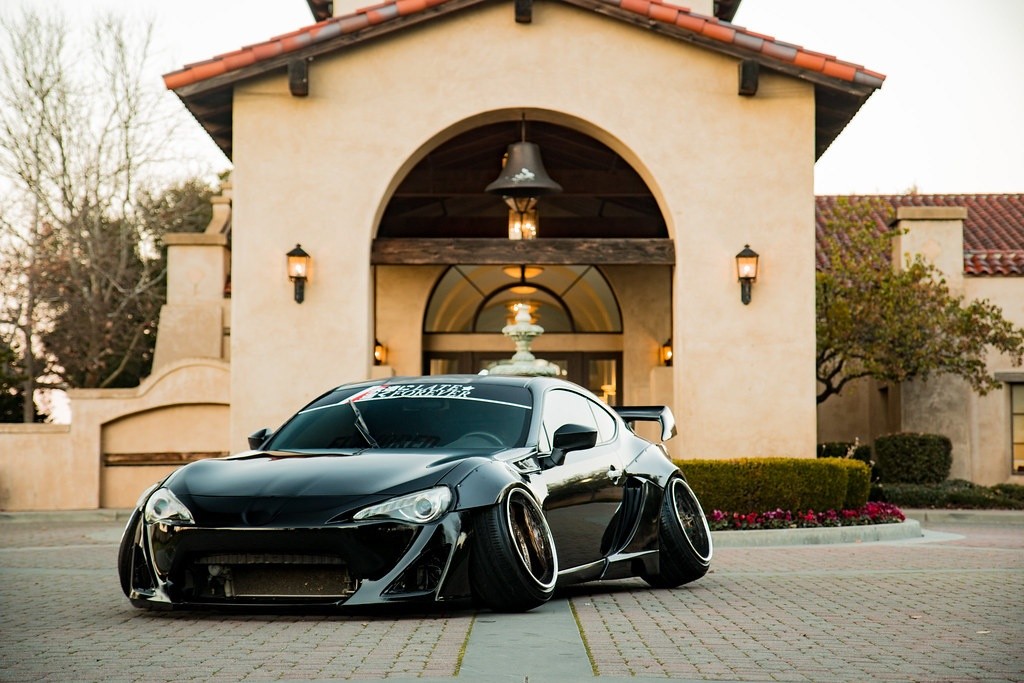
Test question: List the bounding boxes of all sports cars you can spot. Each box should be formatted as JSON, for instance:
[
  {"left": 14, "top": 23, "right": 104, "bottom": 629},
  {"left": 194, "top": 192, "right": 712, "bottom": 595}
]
[{"left": 117, "top": 374, "right": 714, "bottom": 619}]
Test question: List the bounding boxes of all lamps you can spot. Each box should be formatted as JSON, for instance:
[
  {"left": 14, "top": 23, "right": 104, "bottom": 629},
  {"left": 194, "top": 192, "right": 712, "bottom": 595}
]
[
  {"left": 736, "top": 244, "right": 759, "bottom": 304},
  {"left": 486, "top": 122, "right": 565, "bottom": 241},
  {"left": 374, "top": 339, "right": 383, "bottom": 365},
  {"left": 663, "top": 339, "right": 672, "bottom": 366},
  {"left": 286, "top": 244, "right": 310, "bottom": 303}
]
